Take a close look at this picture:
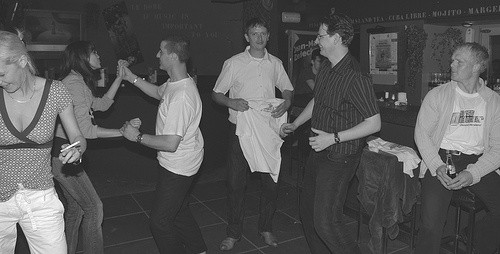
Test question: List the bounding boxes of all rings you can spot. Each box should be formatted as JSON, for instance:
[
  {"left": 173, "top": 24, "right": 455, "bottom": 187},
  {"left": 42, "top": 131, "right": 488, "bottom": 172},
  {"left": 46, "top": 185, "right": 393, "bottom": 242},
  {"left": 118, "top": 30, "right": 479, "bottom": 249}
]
[{"left": 459, "top": 184, "right": 466, "bottom": 189}]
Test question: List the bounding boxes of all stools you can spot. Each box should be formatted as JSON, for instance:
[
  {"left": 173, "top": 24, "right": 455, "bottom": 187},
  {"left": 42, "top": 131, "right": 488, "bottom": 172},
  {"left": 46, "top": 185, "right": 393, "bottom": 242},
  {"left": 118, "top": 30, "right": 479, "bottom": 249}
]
[
  {"left": 354, "top": 134, "right": 421, "bottom": 254},
  {"left": 276, "top": 133, "right": 311, "bottom": 226},
  {"left": 439, "top": 193, "right": 488, "bottom": 254}
]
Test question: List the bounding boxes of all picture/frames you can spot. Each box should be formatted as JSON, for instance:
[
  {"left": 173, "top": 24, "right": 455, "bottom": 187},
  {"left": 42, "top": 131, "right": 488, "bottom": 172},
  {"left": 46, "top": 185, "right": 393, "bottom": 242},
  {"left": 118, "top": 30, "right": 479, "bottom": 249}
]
[{"left": 22, "top": 8, "right": 87, "bottom": 52}]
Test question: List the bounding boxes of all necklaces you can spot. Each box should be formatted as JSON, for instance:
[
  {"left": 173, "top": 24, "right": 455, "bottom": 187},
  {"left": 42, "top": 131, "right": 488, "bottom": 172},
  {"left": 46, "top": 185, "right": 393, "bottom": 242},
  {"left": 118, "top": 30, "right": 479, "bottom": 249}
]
[{"left": 4, "top": 76, "right": 38, "bottom": 104}]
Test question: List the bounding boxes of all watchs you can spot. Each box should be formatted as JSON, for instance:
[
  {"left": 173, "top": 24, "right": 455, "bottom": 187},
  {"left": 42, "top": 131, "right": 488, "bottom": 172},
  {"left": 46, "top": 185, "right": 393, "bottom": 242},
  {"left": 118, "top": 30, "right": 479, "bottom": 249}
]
[{"left": 132, "top": 75, "right": 141, "bottom": 85}]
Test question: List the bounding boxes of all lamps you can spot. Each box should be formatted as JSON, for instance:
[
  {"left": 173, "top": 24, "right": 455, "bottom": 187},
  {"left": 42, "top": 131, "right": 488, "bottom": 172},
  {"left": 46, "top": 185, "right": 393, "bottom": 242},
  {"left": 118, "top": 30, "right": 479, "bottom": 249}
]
[{"left": 281, "top": 10, "right": 301, "bottom": 24}]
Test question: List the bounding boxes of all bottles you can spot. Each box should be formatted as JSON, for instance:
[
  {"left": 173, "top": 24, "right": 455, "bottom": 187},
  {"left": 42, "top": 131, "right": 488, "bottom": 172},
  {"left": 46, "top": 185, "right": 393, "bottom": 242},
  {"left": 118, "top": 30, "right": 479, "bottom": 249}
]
[
  {"left": 392, "top": 92, "right": 395, "bottom": 102},
  {"left": 384, "top": 91, "right": 389, "bottom": 105},
  {"left": 446, "top": 154, "right": 457, "bottom": 180}
]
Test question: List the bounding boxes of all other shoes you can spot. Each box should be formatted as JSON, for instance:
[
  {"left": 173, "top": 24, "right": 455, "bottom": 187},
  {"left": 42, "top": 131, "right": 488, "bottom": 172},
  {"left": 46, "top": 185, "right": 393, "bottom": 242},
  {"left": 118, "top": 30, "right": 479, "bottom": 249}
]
[
  {"left": 258, "top": 231, "right": 278, "bottom": 247},
  {"left": 220, "top": 236, "right": 242, "bottom": 250}
]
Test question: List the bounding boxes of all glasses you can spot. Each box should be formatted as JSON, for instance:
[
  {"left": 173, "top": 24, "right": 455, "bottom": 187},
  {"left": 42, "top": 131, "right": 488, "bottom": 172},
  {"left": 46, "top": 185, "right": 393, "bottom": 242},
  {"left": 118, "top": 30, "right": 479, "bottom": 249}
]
[{"left": 316, "top": 33, "right": 334, "bottom": 40}]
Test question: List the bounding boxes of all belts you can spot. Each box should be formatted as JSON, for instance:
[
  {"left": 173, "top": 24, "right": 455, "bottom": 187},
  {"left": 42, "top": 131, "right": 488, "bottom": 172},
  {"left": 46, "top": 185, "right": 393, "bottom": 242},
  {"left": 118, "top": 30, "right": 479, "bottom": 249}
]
[{"left": 440, "top": 149, "right": 462, "bottom": 157}]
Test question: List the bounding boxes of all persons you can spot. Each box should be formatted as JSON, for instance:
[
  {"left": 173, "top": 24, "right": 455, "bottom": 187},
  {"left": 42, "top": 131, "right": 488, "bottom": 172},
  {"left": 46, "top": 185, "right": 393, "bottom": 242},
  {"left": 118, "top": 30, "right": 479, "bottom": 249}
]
[
  {"left": 280, "top": 12, "right": 381, "bottom": 254},
  {"left": 118, "top": 37, "right": 208, "bottom": 254},
  {"left": 51, "top": 40, "right": 141, "bottom": 254},
  {"left": 212, "top": 17, "right": 294, "bottom": 250},
  {"left": 289, "top": 49, "right": 327, "bottom": 171},
  {"left": 0, "top": 30, "right": 86, "bottom": 254},
  {"left": 414, "top": 43, "right": 500, "bottom": 254}
]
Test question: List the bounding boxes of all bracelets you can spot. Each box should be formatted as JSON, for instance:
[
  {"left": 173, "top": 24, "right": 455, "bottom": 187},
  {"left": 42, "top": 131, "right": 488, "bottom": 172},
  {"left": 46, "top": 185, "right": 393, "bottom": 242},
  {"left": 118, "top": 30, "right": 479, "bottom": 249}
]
[
  {"left": 136, "top": 132, "right": 145, "bottom": 145},
  {"left": 333, "top": 132, "right": 341, "bottom": 146},
  {"left": 74, "top": 147, "right": 83, "bottom": 166}
]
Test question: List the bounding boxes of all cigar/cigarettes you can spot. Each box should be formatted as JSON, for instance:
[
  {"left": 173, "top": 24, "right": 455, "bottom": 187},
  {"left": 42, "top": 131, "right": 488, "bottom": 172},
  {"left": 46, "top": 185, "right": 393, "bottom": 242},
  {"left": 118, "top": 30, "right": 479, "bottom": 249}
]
[{"left": 60, "top": 140, "right": 82, "bottom": 152}]
